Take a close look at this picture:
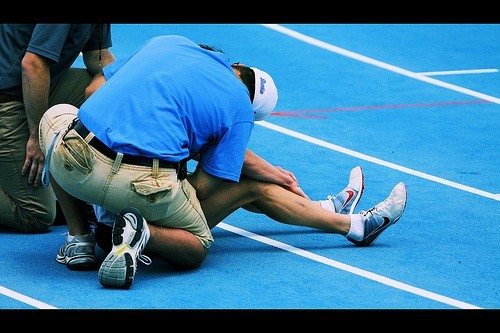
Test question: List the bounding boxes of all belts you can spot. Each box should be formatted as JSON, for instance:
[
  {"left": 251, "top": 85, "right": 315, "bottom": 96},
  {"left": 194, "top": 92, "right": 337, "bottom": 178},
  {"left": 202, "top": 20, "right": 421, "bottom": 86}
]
[{"left": 74, "top": 121, "right": 178, "bottom": 168}]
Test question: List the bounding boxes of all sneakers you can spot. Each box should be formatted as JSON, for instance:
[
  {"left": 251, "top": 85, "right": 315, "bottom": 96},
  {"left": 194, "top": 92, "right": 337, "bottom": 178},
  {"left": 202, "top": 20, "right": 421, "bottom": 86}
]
[
  {"left": 327, "top": 166, "right": 364, "bottom": 214},
  {"left": 98, "top": 207, "right": 152, "bottom": 289},
  {"left": 348, "top": 181, "right": 407, "bottom": 246},
  {"left": 56, "top": 230, "right": 97, "bottom": 269}
]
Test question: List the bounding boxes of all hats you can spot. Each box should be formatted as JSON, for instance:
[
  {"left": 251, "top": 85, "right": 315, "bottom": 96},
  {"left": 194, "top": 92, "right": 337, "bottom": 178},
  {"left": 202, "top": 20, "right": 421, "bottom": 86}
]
[{"left": 250, "top": 67, "right": 278, "bottom": 121}]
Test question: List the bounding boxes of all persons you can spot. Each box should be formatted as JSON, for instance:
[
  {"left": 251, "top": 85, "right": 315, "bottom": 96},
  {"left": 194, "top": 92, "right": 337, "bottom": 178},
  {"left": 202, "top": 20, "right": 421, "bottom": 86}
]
[
  {"left": 0, "top": 24, "right": 114, "bottom": 236},
  {"left": 38, "top": 35, "right": 278, "bottom": 290},
  {"left": 92, "top": 148, "right": 408, "bottom": 248}
]
